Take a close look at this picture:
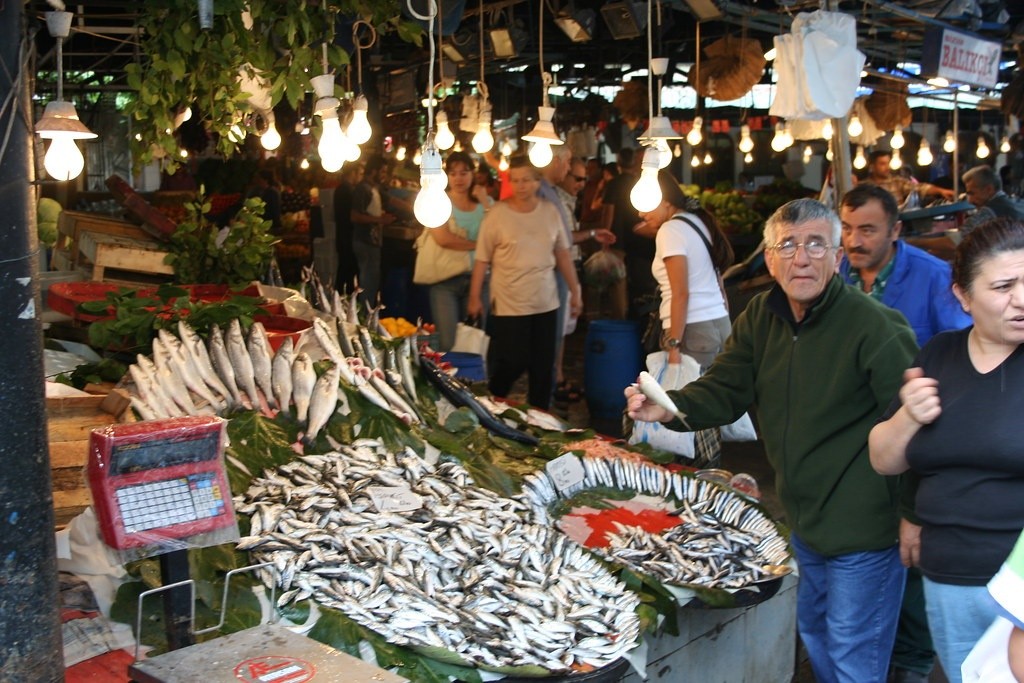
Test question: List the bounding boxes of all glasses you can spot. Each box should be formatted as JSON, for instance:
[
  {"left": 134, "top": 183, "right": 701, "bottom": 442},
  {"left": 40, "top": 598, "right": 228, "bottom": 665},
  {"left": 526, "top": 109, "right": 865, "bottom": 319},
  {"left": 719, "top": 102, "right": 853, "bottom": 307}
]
[{"left": 766, "top": 239, "right": 838, "bottom": 261}]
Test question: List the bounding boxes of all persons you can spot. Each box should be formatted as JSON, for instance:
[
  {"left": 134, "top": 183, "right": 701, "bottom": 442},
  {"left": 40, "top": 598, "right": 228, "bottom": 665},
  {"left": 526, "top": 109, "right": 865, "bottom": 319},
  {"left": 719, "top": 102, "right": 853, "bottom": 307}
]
[
  {"left": 868, "top": 215, "right": 1024, "bottom": 683},
  {"left": 194, "top": 132, "right": 655, "bottom": 414},
  {"left": 624, "top": 166, "right": 733, "bottom": 465},
  {"left": 859, "top": 131, "right": 1024, "bottom": 222},
  {"left": 985, "top": 526, "right": 1023, "bottom": 683},
  {"left": 624, "top": 198, "right": 922, "bottom": 683}
]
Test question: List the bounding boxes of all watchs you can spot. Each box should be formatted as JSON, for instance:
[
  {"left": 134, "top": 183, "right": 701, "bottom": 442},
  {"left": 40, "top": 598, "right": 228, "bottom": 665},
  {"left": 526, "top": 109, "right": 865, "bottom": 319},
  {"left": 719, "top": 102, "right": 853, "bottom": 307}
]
[
  {"left": 667, "top": 337, "right": 683, "bottom": 349},
  {"left": 590, "top": 228, "right": 597, "bottom": 237}
]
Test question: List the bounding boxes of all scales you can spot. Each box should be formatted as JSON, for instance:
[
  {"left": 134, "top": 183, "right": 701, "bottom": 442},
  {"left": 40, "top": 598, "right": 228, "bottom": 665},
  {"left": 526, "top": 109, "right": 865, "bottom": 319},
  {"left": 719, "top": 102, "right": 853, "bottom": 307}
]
[{"left": 86, "top": 415, "right": 410, "bottom": 683}]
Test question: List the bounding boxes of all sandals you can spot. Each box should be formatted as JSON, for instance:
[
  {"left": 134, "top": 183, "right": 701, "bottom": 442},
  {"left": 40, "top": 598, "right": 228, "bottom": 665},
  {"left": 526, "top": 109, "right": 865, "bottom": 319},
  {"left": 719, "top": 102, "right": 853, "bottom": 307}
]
[{"left": 554, "top": 379, "right": 586, "bottom": 402}]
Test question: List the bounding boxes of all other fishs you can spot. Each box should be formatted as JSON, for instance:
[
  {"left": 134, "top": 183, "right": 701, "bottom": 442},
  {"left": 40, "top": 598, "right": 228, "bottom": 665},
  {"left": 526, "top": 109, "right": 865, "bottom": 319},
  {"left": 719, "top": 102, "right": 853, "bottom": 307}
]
[{"left": 124, "top": 264, "right": 793, "bottom": 669}]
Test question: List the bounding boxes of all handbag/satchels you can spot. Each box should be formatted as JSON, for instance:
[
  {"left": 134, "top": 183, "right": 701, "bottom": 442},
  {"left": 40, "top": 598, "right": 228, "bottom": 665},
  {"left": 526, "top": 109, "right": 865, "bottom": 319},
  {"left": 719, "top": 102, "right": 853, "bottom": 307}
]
[
  {"left": 720, "top": 412, "right": 757, "bottom": 443},
  {"left": 629, "top": 351, "right": 701, "bottom": 459},
  {"left": 642, "top": 290, "right": 665, "bottom": 353},
  {"left": 453, "top": 312, "right": 489, "bottom": 364},
  {"left": 414, "top": 210, "right": 473, "bottom": 284},
  {"left": 585, "top": 244, "right": 628, "bottom": 285}
]
